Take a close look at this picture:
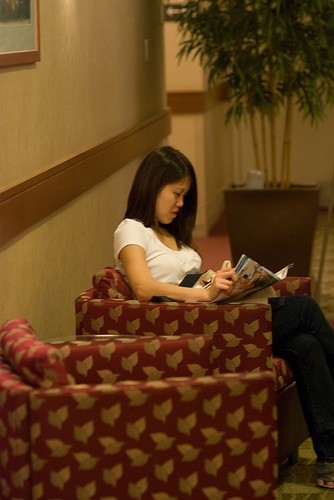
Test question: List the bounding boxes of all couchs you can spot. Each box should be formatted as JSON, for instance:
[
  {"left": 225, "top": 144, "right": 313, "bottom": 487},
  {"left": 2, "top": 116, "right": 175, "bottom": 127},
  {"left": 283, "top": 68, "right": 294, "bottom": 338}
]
[{"left": 0, "top": 264, "right": 312, "bottom": 500}]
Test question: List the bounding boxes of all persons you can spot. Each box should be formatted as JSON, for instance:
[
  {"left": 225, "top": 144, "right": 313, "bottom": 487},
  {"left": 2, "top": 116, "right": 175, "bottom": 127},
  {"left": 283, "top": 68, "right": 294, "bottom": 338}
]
[{"left": 112, "top": 147, "right": 334, "bottom": 488}]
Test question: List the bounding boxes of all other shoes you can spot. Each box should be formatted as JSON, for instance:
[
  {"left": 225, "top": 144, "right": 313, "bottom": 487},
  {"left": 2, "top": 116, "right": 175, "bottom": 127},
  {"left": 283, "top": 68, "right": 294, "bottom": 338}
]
[{"left": 315, "top": 461, "right": 334, "bottom": 490}]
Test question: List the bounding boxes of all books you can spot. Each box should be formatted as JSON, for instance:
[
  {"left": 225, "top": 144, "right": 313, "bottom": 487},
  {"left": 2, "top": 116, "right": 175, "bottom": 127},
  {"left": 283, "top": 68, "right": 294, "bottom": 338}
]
[{"left": 185, "top": 253, "right": 294, "bottom": 304}]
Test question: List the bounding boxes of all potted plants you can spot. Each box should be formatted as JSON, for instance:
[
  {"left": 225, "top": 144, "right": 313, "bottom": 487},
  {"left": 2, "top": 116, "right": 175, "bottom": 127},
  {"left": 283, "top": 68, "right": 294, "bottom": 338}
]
[{"left": 157, "top": 0, "right": 334, "bottom": 276}]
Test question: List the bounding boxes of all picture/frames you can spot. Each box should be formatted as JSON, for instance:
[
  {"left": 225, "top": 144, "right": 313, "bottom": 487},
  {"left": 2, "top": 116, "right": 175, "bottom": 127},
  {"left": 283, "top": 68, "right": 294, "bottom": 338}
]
[{"left": 0, "top": 0, "right": 41, "bottom": 67}]
